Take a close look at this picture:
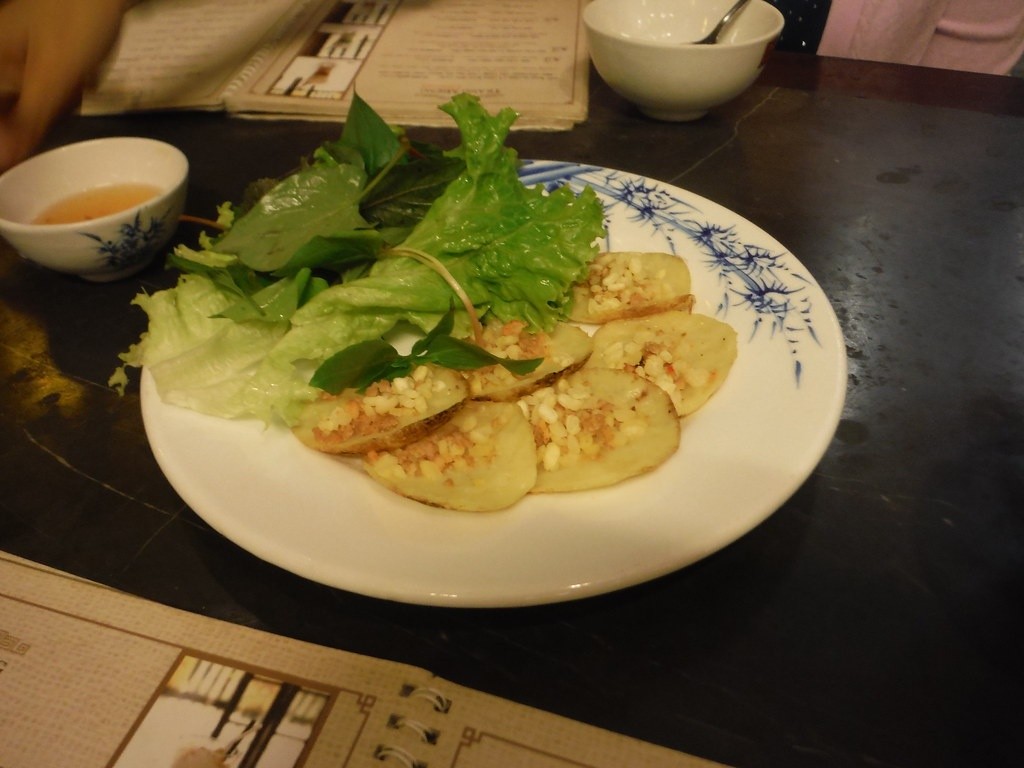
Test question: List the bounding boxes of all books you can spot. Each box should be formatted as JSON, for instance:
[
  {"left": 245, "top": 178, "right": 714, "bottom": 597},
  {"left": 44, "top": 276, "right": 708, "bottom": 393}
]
[
  {"left": 67, "top": 0, "right": 590, "bottom": 134},
  {"left": 0, "top": 549, "right": 730, "bottom": 768}
]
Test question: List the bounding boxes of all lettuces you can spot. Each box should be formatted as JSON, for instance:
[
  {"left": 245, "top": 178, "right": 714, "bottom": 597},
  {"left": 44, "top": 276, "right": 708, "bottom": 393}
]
[{"left": 109, "top": 94, "right": 611, "bottom": 425}]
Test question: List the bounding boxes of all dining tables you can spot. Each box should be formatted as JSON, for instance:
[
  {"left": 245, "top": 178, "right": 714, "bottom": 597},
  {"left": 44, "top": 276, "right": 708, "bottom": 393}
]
[{"left": 2, "top": 49, "right": 1023, "bottom": 767}]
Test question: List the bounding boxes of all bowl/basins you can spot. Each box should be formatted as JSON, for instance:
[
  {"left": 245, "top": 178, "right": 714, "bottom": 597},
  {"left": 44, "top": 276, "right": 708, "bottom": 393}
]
[
  {"left": 582, "top": 0, "right": 786, "bottom": 121},
  {"left": 0, "top": 136, "right": 188, "bottom": 283}
]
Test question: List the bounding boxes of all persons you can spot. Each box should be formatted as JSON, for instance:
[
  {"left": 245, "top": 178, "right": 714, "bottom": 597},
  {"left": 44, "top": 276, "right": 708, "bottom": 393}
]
[{"left": 0, "top": 0, "right": 132, "bottom": 169}]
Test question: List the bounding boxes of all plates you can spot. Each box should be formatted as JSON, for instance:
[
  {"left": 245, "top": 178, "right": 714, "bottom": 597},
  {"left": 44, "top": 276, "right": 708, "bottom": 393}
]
[{"left": 141, "top": 159, "right": 848, "bottom": 607}]
